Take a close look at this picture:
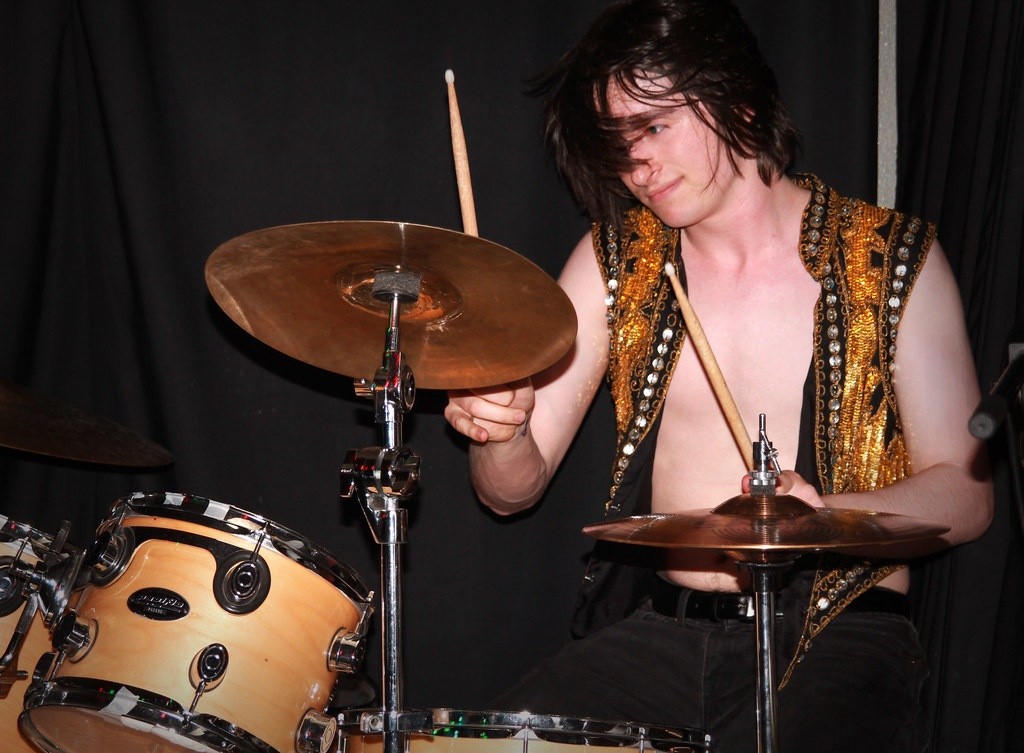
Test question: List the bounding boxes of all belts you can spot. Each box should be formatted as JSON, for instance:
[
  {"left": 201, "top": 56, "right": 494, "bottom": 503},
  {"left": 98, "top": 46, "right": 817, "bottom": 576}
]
[{"left": 650, "top": 573, "right": 911, "bottom": 624}]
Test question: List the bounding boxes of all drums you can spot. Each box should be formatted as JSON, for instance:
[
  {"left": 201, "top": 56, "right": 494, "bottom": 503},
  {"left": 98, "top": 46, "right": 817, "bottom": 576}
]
[
  {"left": 334, "top": 703, "right": 713, "bottom": 753},
  {"left": 17, "top": 484, "right": 376, "bottom": 753},
  {"left": 0, "top": 512, "right": 88, "bottom": 753}
]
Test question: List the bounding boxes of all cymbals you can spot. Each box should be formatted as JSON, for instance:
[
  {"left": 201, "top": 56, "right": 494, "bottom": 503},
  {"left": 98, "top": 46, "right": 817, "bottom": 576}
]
[
  {"left": 202, "top": 215, "right": 579, "bottom": 396},
  {"left": 0, "top": 376, "right": 179, "bottom": 469},
  {"left": 579, "top": 492, "right": 951, "bottom": 565}
]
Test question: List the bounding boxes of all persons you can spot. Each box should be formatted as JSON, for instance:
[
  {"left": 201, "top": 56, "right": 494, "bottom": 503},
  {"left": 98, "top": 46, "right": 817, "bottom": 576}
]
[{"left": 442, "top": 0, "right": 995, "bottom": 753}]
